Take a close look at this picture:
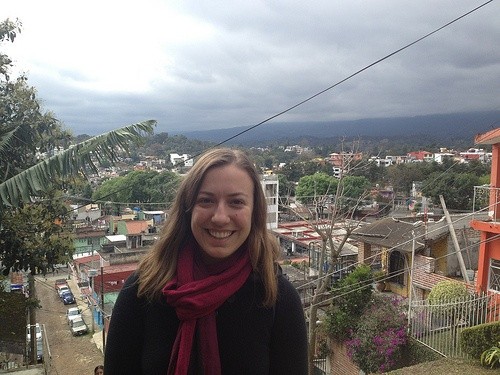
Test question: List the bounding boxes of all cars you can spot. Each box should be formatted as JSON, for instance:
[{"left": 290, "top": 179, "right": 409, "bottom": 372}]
[
  {"left": 26, "top": 323, "right": 43, "bottom": 362},
  {"left": 54, "top": 278, "right": 88, "bottom": 336}
]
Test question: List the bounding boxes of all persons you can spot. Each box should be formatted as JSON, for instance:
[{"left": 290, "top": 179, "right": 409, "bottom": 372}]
[
  {"left": 103, "top": 147, "right": 309, "bottom": 375},
  {"left": 94, "top": 365, "right": 104, "bottom": 375}
]
